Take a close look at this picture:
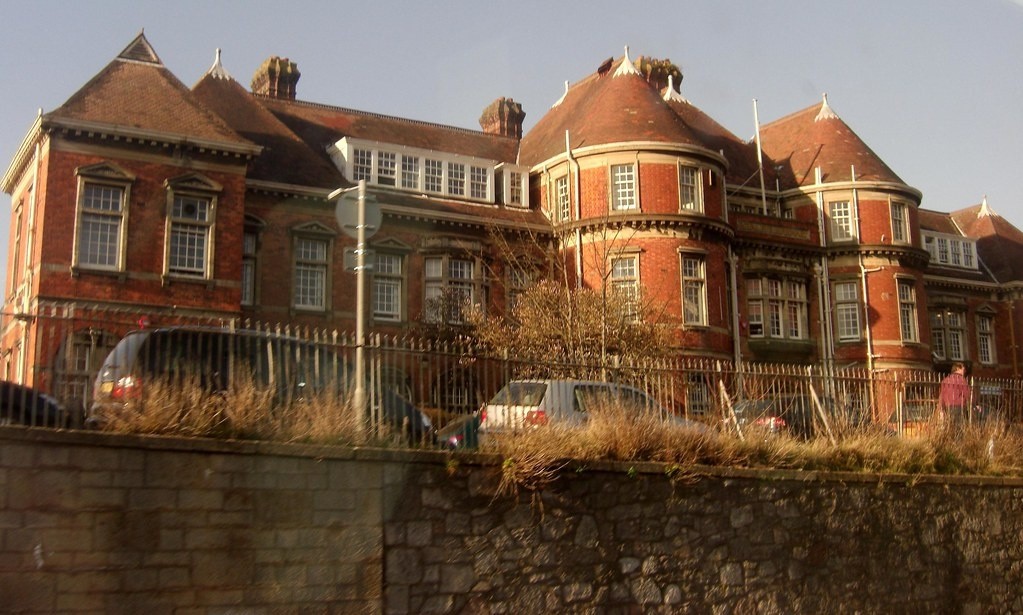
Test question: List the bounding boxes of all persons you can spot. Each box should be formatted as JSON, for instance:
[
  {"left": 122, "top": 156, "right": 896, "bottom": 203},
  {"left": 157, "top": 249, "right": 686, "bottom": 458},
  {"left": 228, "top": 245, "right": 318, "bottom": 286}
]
[{"left": 939, "top": 363, "right": 981, "bottom": 417}]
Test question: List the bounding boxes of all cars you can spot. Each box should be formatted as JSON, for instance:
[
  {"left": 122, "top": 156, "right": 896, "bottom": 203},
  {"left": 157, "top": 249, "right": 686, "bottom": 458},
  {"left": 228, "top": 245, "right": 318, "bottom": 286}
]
[
  {"left": 478, "top": 379, "right": 721, "bottom": 454},
  {"left": 720, "top": 393, "right": 883, "bottom": 443},
  {"left": 85, "top": 327, "right": 438, "bottom": 451},
  {"left": 888, "top": 399, "right": 1015, "bottom": 442},
  {"left": 437, "top": 414, "right": 481, "bottom": 453},
  {"left": 0, "top": 381, "right": 76, "bottom": 430}
]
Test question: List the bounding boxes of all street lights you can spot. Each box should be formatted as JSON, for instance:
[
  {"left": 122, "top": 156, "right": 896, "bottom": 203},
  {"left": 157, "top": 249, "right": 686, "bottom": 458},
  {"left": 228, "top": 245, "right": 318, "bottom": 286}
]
[{"left": 327, "top": 180, "right": 367, "bottom": 446}]
